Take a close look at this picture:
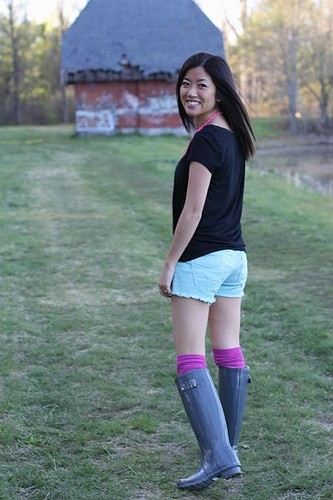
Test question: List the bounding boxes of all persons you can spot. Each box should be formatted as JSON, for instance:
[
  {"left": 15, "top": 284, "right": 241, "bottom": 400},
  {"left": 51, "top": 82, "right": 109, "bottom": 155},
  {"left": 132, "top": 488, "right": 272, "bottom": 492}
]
[{"left": 156, "top": 52, "right": 258, "bottom": 494}]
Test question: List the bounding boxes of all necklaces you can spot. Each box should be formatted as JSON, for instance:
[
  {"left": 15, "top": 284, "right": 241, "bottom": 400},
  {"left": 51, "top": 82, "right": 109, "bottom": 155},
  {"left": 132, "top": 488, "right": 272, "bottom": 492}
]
[{"left": 194, "top": 111, "right": 223, "bottom": 132}]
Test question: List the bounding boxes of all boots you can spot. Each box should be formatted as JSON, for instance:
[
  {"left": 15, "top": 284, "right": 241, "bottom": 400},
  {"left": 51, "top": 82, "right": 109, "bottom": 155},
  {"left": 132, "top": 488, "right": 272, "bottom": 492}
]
[
  {"left": 218, "top": 365, "right": 250, "bottom": 454},
  {"left": 172, "top": 367, "right": 245, "bottom": 491}
]
